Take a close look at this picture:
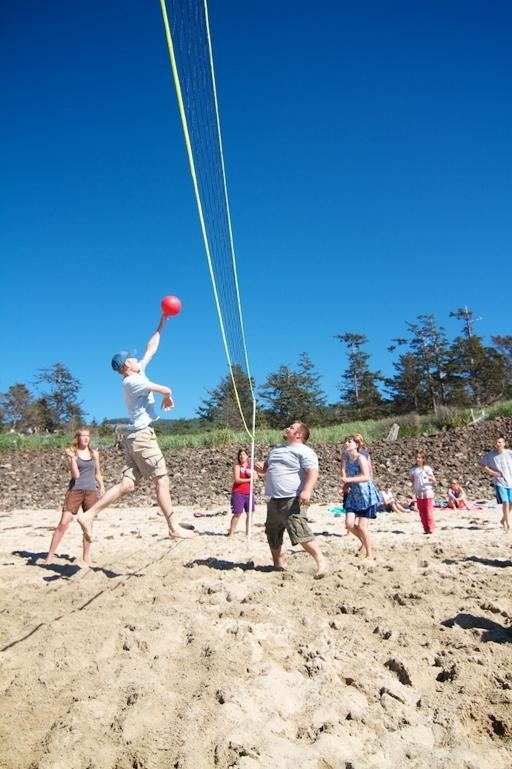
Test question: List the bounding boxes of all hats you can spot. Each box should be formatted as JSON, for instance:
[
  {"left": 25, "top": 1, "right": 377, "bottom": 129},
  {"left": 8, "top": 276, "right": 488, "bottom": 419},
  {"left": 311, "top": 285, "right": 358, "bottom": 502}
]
[{"left": 111, "top": 350, "right": 136, "bottom": 371}]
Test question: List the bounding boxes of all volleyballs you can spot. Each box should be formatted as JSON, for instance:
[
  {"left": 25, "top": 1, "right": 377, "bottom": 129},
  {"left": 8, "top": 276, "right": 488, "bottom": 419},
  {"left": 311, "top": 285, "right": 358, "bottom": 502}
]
[{"left": 160, "top": 295, "right": 180, "bottom": 315}]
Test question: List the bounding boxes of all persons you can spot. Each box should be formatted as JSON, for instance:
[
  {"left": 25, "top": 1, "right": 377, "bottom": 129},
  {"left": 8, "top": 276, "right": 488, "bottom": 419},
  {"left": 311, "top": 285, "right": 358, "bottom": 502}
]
[
  {"left": 409, "top": 452, "right": 437, "bottom": 535},
  {"left": 341, "top": 433, "right": 372, "bottom": 534},
  {"left": 447, "top": 478, "right": 472, "bottom": 510},
  {"left": 479, "top": 438, "right": 512, "bottom": 532},
  {"left": 338, "top": 435, "right": 370, "bottom": 558},
  {"left": 374, "top": 481, "right": 418, "bottom": 513},
  {"left": 77, "top": 314, "right": 195, "bottom": 543},
  {"left": 246, "top": 420, "right": 327, "bottom": 579},
  {"left": 225, "top": 449, "right": 258, "bottom": 537},
  {"left": 43, "top": 428, "right": 105, "bottom": 564}
]
